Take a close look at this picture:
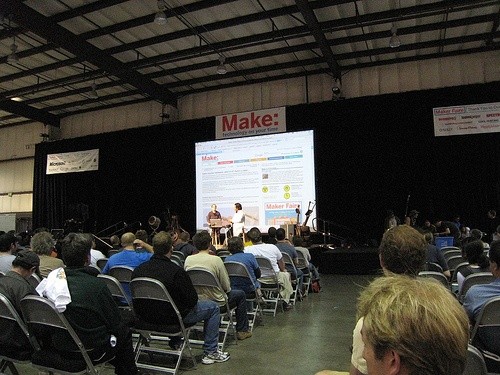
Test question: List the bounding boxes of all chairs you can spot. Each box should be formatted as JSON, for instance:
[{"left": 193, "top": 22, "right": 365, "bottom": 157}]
[
  {"left": 439, "top": 246, "right": 500, "bottom": 375},
  {"left": 1, "top": 249, "right": 312, "bottom": 375},
  {"left": 419, "top": 271, "right": 449, "bottom": 288}
]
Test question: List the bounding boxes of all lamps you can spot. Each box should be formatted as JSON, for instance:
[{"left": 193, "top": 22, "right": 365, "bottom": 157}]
[
  {"left": 154, "top": 1, "right": 168, "bottom": 25},
  {"left": 160, "top": 112, "right": 169, "bottom": 119},
  {"left": 88, "top": 83, "right": 98, "bottom": 98},
  {"left": 216, "top": 56, "right": 227, "bottom": 74},
  {"left": 389, "top": 27, "right": 400, "bottom": 47},
  {"left": 332, "top": 87, "right": 341, "bottom": 95},
  {"left": 149, "top": 216, "right": 161, "bottom": 227},
  {"left": 39, "top": 133, "right": 48, "bottom": 138},
  {"left": 7, "top": 44, "right": 18, "bottom": 62}
]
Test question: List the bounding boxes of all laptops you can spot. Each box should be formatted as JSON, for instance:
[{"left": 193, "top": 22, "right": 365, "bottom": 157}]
[{"left": 209, "top": 219, "right": 225, "bottom": 225}]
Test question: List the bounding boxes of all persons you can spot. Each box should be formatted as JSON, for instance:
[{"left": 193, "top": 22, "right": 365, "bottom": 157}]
[
  {"left": 0, "top": 251, "right": 43, "bottom": 326},
  {"left": 434, "top": 219, "right": 461, "bottom": 246},
  {"left": 403, "top": 209, "right": 437, "bottom": 233},
  {"left": 134, "top": 231, "right": 230, "bottom": 364},
  {"left": 384, "top": 209, "right": 400, "bottom": 229},
  {"left": 243, "top": 228, "right": 294, "bottom": 311},
  {"left": 184, "top": 230, "right": 252, "bottom": 340},
  {"left": 293, "top": 235, "right": 321, "bottom": 290},
  {"left": 110, "top": 230, "right": 218, "bottom": 260},
  {"left": 454, "top": 209, "right": 500, "bottom": 330},
  {"left": 72, "top": 202, "right": 82, "bottom": 219},
  {"left": 62, "top": 232, "right": 138, "bottom": 375},
  {"left": 378, "top": 224, "right": 428, "bottom": 277},
  {"left": 224, "top": 237, "right": 261, "bottom": 299},
  {"left": 266, "top": 227, "right": 305, "bottom": 300},
  {"left": 100, "top": 232, "right": 155, "bottom": 304},
  {"left": 0, "top": 228, "right": 120, "bottom": 274},
  {"left": 162, "top": 208, "right": 178, "bottom": 230},
  {"left": 352, "top": 273, "right": 470, "bottom": 375}
]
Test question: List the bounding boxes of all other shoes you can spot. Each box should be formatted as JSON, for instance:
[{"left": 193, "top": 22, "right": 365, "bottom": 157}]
[{"left": 283, "top": 303, "right": 291, "bottom": 310}]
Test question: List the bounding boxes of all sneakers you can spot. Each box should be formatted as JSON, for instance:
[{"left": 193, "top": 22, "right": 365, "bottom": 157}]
[
  {"left": 168, "top": 337, "right": 184, "bottom": 350},
  {"left": 202, "top": 350, "right": 230, "bottom": 364},
  {"left": 236, "top": 330, "right": 252, "bottom": 340}
]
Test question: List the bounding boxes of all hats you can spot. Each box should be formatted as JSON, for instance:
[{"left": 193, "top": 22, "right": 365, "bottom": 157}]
[
  {"left": 409, "top": 210, "right": 420, "bottom": 215},
  {"left": 16, "top": 250, "right": 40, "bottom": 273}
]
